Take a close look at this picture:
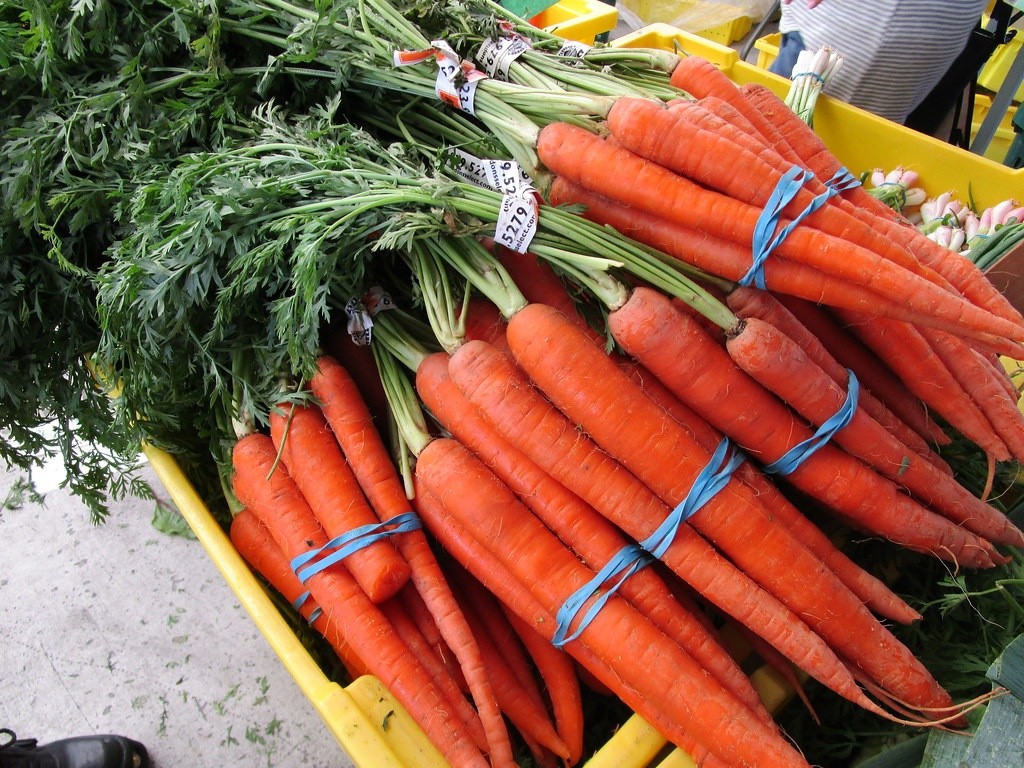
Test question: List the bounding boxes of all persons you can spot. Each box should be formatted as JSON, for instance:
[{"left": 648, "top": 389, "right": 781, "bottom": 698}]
[{"left": 769, "top": 0, "right": 988, "bottom": 124}]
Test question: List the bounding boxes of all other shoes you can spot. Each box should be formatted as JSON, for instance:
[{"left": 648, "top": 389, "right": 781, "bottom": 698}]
[{"left": 0, "top": 727, "right": 151, "bottom": 768}]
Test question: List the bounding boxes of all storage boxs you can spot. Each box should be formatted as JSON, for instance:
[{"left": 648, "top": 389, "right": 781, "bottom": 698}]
[{"left": 91, "top": 0, "right": 1024, "bottom": 767}]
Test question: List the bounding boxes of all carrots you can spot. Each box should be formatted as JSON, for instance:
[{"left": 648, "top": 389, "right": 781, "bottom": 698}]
[{"left": 228, "top": 55, "right": 1024, "bottom": 768}]
